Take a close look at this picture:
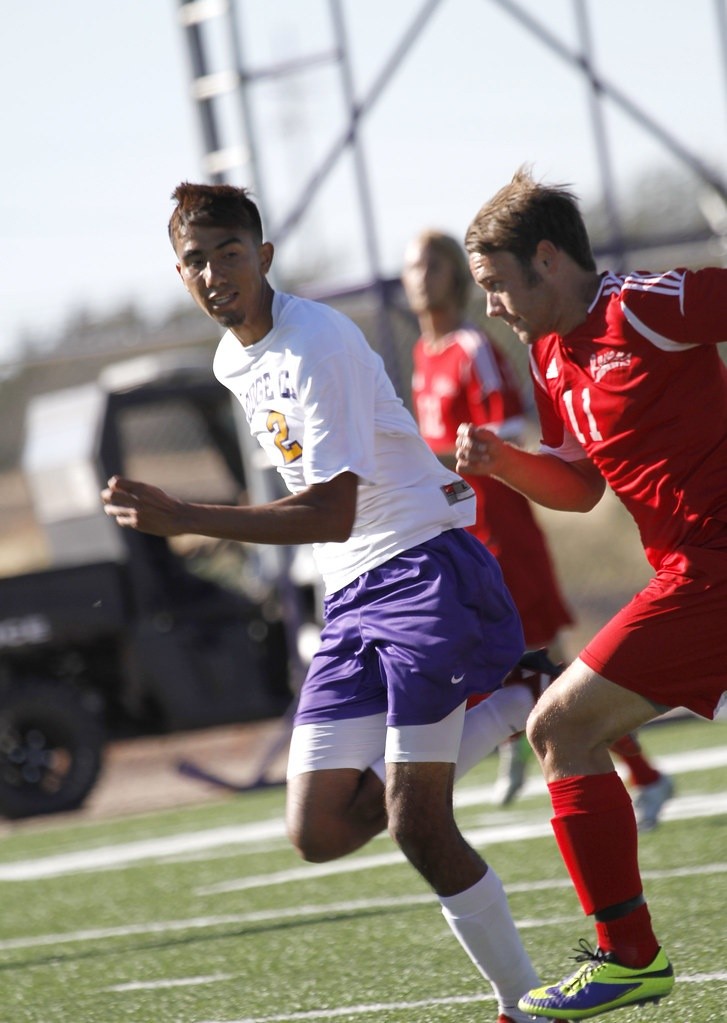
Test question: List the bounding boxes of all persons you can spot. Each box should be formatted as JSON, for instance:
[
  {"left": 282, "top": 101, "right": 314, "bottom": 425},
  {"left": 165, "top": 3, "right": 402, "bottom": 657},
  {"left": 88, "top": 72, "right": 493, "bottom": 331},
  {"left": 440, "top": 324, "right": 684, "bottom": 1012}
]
[
  {"left": 101, "top": 183, "right": 570, "bottom": 1023},
  {"left": 456, "top": 196, "right": 727, "bottom": 1023},
  {"left": 396, "top": 229, "right": 673, "bottom": 828}
]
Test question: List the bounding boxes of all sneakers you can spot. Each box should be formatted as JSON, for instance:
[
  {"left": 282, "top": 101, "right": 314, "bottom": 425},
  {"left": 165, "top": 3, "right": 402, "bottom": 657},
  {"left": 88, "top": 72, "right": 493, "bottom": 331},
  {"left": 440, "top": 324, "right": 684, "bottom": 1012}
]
[
  {"left": 494, "top": 733, "right": 530, "bottom": 807},
  {"left": 517, "top": 938, "right": 675, "bottom": 1020},
  {"left": 631, "top": 775, "right": 672, "bottom": 828},
  {"left": 502, "top": 649, "right": 568, "bottom": 703}
]
